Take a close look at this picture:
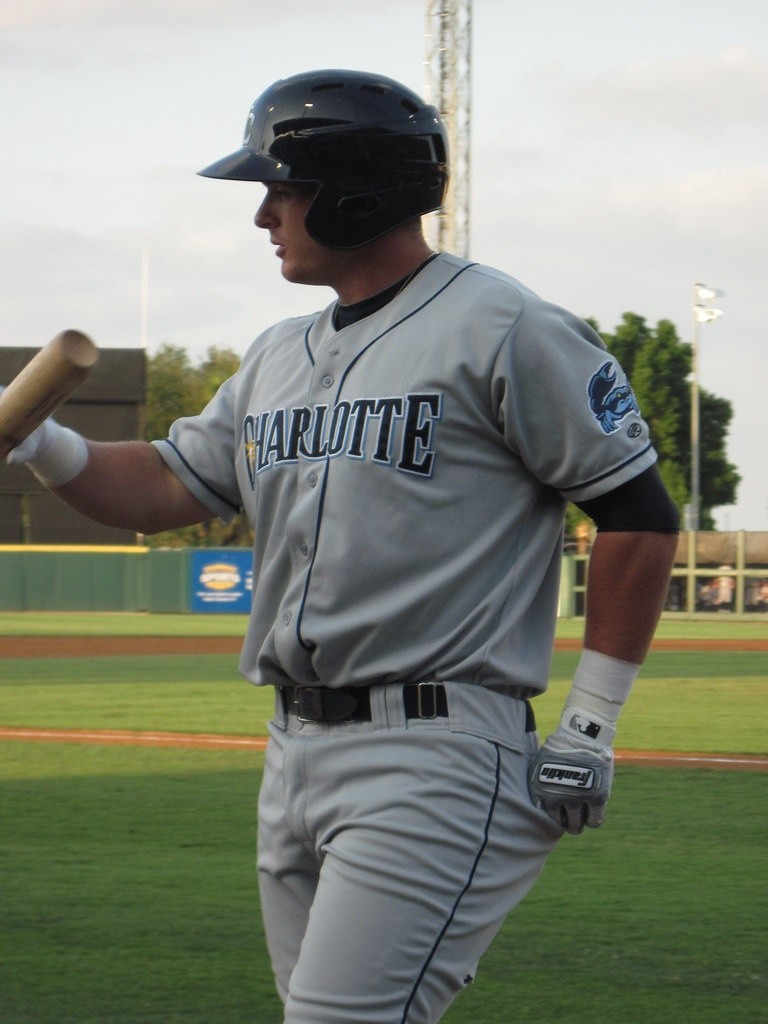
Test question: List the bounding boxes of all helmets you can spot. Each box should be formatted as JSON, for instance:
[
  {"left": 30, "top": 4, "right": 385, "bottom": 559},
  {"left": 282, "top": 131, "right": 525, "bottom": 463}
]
[{"left": 196, "top": 69, "right": 451, "bottom": 250}]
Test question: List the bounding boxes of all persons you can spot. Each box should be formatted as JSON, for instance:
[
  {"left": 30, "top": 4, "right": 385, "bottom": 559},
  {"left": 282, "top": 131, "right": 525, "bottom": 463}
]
[{"left": 0, "top": 73, "right": 679, "bottom": 1024}]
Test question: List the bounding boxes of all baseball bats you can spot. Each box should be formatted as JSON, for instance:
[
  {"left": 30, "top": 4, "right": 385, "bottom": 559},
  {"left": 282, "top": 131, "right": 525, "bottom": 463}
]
[{"left": 0, "top": 328, "right": 102, "bottom": 455}]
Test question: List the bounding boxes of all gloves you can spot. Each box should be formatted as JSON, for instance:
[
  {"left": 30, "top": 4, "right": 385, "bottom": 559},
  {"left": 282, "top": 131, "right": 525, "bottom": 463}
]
[
  {"left": 528, "top": 709, "right": 614, "bottom": 836},
  {"left": 0, "top": 385, "right": 47, "bottom": 466}
]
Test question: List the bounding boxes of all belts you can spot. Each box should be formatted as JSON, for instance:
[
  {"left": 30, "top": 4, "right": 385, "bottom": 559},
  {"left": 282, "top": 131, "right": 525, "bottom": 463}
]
[{"left": 281, "top": 683, "right": 448, "bottom": 725}]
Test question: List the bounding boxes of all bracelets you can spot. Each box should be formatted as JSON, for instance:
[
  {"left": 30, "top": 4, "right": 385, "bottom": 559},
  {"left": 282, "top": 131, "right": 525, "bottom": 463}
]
[{"left": 563, "top": 705, "right": 616, "bottom": 750}]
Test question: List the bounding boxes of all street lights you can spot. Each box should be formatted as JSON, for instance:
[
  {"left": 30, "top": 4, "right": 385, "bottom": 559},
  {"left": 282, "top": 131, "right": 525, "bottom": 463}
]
[{"left": 686, "top": 282, "right": 724, "bottom": 615}]
